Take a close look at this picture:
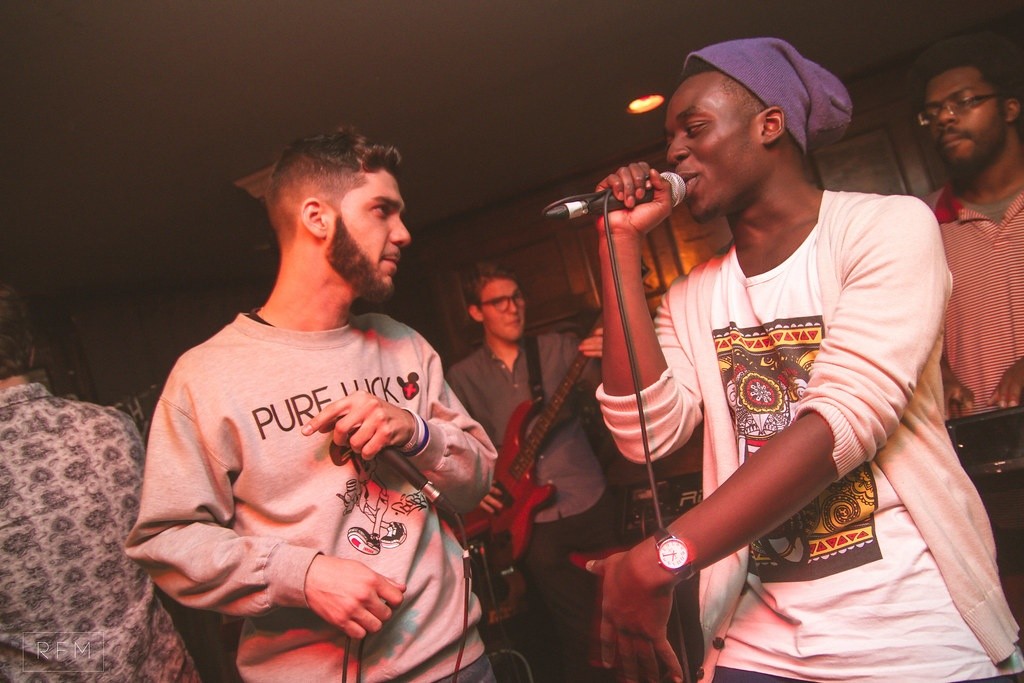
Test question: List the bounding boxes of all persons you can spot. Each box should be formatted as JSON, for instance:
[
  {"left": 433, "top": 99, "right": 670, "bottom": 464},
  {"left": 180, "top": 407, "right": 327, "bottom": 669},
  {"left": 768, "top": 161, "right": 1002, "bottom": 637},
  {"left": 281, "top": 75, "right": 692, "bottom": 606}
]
[
  {"left": 566, "top": 38, "right": 1024, "bottom": 683},
  {"left": 124, "top": 128, "right": 501, "bottom": 683},
  {"left": 1, "top": 283, "right": 203, "bottom": 682},
  {"left": 918, "top": 32, "right": 1024, "bottom": 418},
  {"left": 446, "top": 263, "right": 605, "bottom": 631}
]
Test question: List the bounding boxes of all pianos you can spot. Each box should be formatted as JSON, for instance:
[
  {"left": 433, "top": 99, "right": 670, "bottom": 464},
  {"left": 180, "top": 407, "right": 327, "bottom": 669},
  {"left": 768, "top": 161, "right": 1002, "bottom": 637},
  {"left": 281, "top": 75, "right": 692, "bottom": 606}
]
[{"left": 943, "top": 400, "right": 1023, "bottom": 481}]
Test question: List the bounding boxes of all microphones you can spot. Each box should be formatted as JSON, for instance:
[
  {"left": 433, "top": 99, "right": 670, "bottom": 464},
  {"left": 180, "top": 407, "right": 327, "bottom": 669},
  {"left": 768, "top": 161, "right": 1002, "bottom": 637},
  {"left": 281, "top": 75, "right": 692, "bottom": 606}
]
[
  {"left": 345, "top": 423, "right": 454, "bottom": 517},
  {"left": 538, "top": 171, "right": 689, "bottom": 221}
]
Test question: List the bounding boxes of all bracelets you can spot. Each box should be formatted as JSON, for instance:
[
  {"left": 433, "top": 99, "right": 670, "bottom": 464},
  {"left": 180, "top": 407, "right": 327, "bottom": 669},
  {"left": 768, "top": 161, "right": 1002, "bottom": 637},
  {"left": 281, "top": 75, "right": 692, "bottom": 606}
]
[{"left": 400, "top": 415, "right": 418, "bottom": 451}]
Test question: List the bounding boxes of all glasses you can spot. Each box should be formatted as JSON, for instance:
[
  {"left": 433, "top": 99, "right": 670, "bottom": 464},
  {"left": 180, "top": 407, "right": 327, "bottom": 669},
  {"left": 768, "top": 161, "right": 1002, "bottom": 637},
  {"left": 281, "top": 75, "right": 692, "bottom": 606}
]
[
  {"left": 917, "top": 93, "right": 1007, "bottom": 128},
  {"left": 479, "top": 292, "right": 527, "bottom": 311}
]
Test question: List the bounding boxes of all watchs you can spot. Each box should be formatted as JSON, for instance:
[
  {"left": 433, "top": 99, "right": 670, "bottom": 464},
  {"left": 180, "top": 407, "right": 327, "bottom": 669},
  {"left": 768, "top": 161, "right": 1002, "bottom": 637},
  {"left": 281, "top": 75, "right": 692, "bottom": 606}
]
[{"left": 653, "top": 529, "right": 694, "bottom": 580}]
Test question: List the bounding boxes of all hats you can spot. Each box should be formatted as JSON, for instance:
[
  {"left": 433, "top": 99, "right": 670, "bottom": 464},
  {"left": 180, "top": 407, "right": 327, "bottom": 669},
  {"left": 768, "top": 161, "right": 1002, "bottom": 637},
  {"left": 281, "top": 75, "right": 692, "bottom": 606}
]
[{"left": 685, "top": 37, "right": 852, "bottom": 157}]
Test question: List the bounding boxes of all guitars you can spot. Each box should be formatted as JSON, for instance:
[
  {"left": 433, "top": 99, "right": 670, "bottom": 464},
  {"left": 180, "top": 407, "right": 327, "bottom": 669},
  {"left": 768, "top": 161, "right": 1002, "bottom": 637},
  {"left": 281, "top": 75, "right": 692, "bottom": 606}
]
[{"left": 445, "top": 256, "right": 653, "bottom": 574}]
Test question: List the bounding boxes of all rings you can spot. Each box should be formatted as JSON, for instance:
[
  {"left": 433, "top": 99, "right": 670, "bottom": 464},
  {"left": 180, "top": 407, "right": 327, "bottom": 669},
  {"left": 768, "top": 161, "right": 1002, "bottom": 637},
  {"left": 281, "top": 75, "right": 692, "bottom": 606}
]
[{"left": 586, "top": 559, "right": 596, "bottom": 571}]
